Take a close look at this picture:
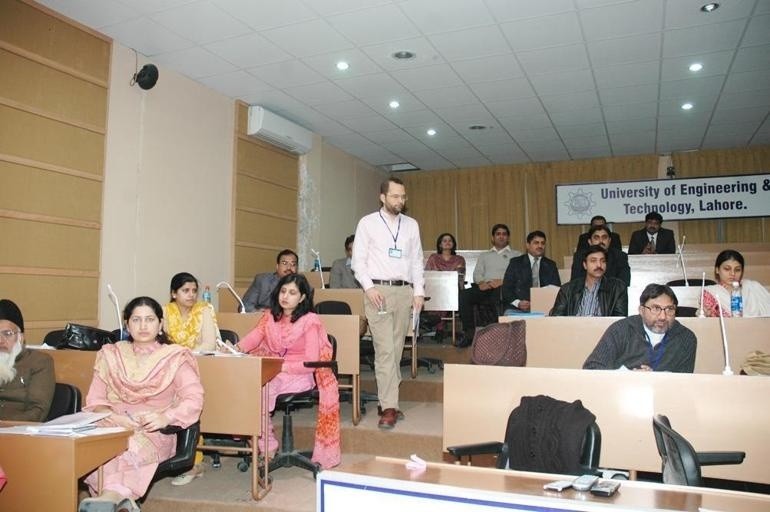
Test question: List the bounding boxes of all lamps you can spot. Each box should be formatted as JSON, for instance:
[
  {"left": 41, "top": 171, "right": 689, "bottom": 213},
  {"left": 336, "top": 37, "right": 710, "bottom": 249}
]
[
  {"left": 395, "top": 50, "right": 413, "bottom": 59},
  {"left": 700, "top": 1, "right": 720, "bottom": 14}
]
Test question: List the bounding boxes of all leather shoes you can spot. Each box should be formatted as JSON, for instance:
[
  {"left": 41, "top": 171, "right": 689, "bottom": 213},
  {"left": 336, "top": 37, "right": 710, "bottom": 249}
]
[
  {"left": 78, "top": 492, "right": 119, "bottom": 510},
  {"left": 116, "top": 498, "right": 141, "bottom": 512},
  {"left": 454, "top": 335, "right": 473, "bottom": 347},
  {"left": 378, "top": 405, "right": 405, "bottom": 419},
  {"left": 376, "top": 408, "right": 398, "bottom": 429}
]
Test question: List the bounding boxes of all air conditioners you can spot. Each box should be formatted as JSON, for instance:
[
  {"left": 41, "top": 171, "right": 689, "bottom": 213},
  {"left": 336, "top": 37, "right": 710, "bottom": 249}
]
[{"left": 248, "top": 106, "right": 313, "bottom": 156}]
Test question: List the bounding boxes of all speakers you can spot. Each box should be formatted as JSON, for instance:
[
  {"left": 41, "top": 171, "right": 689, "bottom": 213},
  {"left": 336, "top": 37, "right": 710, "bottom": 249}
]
[{"left": 133, "top": 63, "right": 160, "bottom": 91}]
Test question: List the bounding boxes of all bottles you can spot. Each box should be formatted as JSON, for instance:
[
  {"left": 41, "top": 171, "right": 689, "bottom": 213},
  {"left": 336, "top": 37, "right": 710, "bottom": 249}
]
[
  {"left": 730, "top": 281, "right": 744, "bottom": 319},
  {"left": 202, "top": 284, "right": 211, "bottom": 305},
  {"left": 315, "top": 251, "right": 322, "bottom": 272}
]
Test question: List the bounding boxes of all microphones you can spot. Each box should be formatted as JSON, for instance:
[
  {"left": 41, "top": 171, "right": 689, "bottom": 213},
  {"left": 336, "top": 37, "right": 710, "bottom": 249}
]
[
  {"left": 697, "top": 271, "right": 709, "bottom": 319},
  {"left": 106, "top": 282, "right": 123, "bottom": 343},
  {"left": 676, "top": 236, "right": 685, "bottom": 269},
  {"left": 217, "top": 279, "right": 247, "bottom": 315},
  {"left": 308, "top": 247, "right": 324, "bottom": 292},
  {"left": 677, "top": 244, "right": 691, "bottom": 288},
  {"left": 714, "top": 292, "right": 736, "bottom": 377}
]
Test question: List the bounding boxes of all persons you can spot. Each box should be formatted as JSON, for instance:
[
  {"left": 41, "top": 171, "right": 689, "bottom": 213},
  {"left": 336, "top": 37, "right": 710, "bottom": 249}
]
[
  {"left": 549, "top": 246, "right": 628, "bottom": 317},
  {"left": 695, "top": 250, "right": 770, "bottom": 317},
  {"left": 582, "top": 284, "right": 697, "bottom": 374},
  {"left": 238, "top": 249, "right": 299, "bottom": 313},
  {"left": 424, "top": 233, "right": 466, "bottom": 345},
  {"left": 0, "top": 299, "right": 56, "bottom": 422},
  {"left": 577, "top": 215, "right": 622, "bottom": 251},
  {"left": 79, "top": 296, "right": 205, "bottom": 512},
  {"left": 628, "top": 212, "right": 676, "bottom": 254},
  {"left": 216, "top": 273, "right": 341, "bottom": 470},
  {"left": 502, "top": 230, "right": 561, "bottom": 312},
  {"left": 572, "top": 225, "right": 631, "bottom": 286},
  {"left": 351, "top": 177, "right": 425, "bottom": 430},
  {"left": 329, "top": 235, "right": 364, "bottom": 288},
  {"left": 161, "top": 272, "right": 223, "bottom": 486},
  {"left": 455, "top": 224, "right": 523, "bottom": 348}
]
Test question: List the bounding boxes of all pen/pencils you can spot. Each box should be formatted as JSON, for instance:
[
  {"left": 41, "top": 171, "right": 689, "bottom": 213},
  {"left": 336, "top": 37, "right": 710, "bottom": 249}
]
[
  {"left": 217, "top": 338, "right": 237, "bottom": 357},
  {"left": 124, "top": 411, "right": 138, "bottom": 426}
]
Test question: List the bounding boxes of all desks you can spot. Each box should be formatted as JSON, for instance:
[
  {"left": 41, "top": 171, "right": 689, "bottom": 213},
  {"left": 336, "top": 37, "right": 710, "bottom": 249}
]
[
  {"left": 422, "top": 249, "right": 491, "bottom": 289},
  {"left": 0, "top": 417, "right": 135, "bottom": 512},
  {"left": 216, "top": 285, "right": 364, "bottom": 315},
  {"left": 304, "top": 270, "right": 419, "bottom": 381},
  {"left": 564, "top": 251, "right": 770, "bottom": 266},
  {"left": 497, "top": 315, "right": 770, "bottom": 375},
  {"left": 42, "top": 348, "right": 286, "bottom": 501},
  {"left": 529, "top": 286, "right": 770, "bottom": 315},
  {"left": 216, "top": 312, "right": 361, "bottom": 426},
  {"left": 422, "top": 271, "right": 459, "bottom": 344},
  {"left": 622, "top": 243, "right": 769, "bottom": 251},
  {"left": 316, "top": 463, "right": 769, "bottom": 510},
  {"left": 558, "top": 265, "right": 770, "bottom": 285}
]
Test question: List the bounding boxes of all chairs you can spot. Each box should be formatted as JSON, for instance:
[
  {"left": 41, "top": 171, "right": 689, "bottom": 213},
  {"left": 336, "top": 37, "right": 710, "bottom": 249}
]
[
  {"left": 155, "top": 422, "right": 201, "bottom": 487},
  {"left": 48, "top": 383, "right": 81, "bottom": 418},
  {"left": 238, "top": 335, "right": 339, "bottom": 478},
  {"left": 653, "top": 415, "right": 746, "bottom": 488},
  {"left": 447, "top": 397, "right": 601, "bottom": 476},
  {"left": 206, "top": 330, "right": 240, "bottom": 467}
]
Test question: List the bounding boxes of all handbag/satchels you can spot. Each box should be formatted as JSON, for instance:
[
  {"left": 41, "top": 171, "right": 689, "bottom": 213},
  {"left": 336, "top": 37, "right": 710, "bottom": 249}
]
[
  {"left": 470, "top": 317, "right": 527, "bottom": 368},
  {"left": 56, "top": 322, "right": 119, "bottom": 351}
]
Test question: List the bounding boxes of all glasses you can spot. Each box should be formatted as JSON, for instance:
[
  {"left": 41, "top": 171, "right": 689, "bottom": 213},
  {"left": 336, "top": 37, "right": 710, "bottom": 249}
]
[
  {"left": 640, "top": 303, "right": 678, "bottom": 317},
  {"left": 276, "top": 261, "right": 297, "bottom": 269},
  {"left": 1, "top": 331, "right": 20, "bottom": 339},
  {"left": 384, "top": 192, "right": 410, "bottom": 203}
]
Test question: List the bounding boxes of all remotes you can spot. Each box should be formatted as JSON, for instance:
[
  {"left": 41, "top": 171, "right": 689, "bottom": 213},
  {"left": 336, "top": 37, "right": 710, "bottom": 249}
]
[
  {"left": 573, "top": 473, "right": 599, "bottom": 492},
  {"left": 591, "top": 478, "right": 621, "bottom": 497}
]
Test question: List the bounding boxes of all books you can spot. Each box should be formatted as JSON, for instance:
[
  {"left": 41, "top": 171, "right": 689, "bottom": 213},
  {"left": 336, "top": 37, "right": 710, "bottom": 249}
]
[{"left": 35, "top": 411, "right": 111, "bottom": 432}]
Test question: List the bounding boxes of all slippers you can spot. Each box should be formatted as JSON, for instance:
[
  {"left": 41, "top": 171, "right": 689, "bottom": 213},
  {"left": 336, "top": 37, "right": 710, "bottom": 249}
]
[{"left": 170, "top": 473, "right": 205, "bottom": 488}]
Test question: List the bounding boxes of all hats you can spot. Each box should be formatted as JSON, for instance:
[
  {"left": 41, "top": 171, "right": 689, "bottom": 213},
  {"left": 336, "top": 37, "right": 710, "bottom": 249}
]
[{"left": 0, "top": 298, "right": 26, "bottom": 331}]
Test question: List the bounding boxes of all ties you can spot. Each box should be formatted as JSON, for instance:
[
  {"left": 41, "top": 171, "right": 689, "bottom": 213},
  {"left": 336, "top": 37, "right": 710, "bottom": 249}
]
[
  {"left": 530, "top": 256, "right": 541, "bottom": 288},
  {"left": 649, "top": 235, "right": 655, "bottom": 249}
]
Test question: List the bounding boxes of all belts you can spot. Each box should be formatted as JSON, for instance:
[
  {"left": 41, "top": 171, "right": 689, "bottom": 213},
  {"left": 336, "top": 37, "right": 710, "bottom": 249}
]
[{"left": 370, "top": 279, "right": 411, "bottom": 287}]
